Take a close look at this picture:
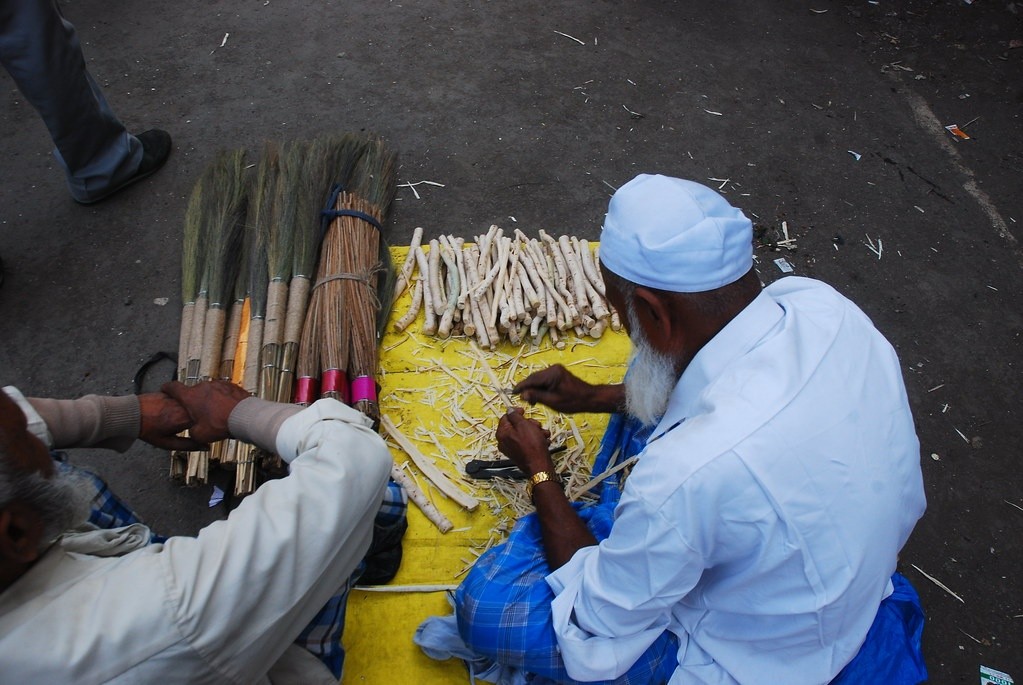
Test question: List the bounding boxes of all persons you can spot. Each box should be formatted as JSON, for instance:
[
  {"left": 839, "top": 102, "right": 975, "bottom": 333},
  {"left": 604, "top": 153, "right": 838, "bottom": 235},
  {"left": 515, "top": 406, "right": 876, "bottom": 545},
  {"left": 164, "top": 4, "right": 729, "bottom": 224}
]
[
  {"left": 455, "top": 172, "right": 929, "bottom": 685},
  {"left": 1, "top": 378, "right": 392, "bottom": 684},
  {"left": 0, "top": 0, "right": 171, "bottom": 205}
]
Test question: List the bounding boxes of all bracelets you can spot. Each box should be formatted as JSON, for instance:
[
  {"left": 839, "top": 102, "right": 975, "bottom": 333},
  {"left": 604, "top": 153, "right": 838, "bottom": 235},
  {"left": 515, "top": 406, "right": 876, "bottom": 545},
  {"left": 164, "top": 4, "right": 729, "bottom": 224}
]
[{"left": 526, "top": 471, "right": 565, "bottom": 506}]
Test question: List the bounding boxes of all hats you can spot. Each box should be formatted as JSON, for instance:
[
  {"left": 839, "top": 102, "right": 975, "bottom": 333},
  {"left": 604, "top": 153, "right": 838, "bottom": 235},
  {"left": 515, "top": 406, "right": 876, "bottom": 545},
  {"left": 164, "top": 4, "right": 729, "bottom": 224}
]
[{"left": 599, "top": 173, "right": 754, "bottom": 293}]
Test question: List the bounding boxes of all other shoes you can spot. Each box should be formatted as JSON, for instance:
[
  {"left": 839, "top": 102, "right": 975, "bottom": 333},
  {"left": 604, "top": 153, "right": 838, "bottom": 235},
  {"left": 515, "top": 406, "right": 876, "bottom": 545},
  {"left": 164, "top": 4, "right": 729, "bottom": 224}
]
[
  {"left": 70, "top": 129, "right": 173, "bottom": 206},
  {"left": 357, "top": 512, "right": 408, "bottom": 586}
]
